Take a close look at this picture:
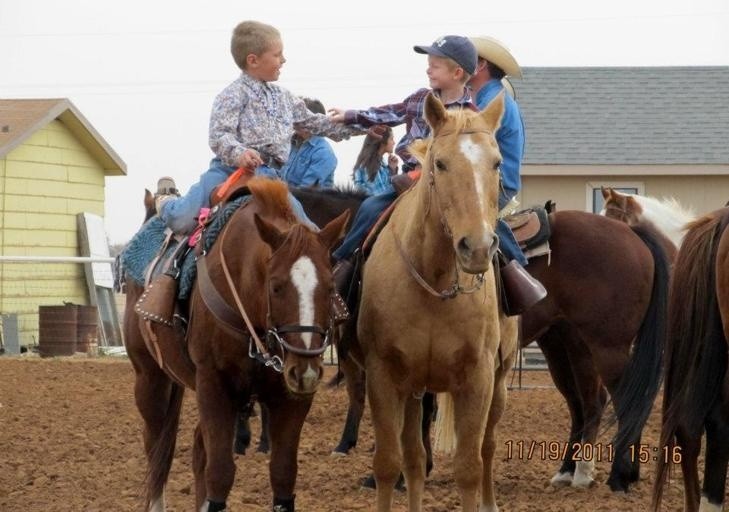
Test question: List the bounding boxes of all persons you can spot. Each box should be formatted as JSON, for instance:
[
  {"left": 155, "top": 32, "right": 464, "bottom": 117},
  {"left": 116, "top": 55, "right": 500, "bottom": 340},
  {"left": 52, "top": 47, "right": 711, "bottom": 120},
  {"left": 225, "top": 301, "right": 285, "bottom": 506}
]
[
  {"left": 328, "top": 35, "right": 528, "bottom": 268},
  {"left": 467, "top": 36, "right": 525, "bottom": 212},
  {"left": 352, "top": 124, "right": 399, "bottom": 196},
  {"left": 154, "top": 20, "right": 390, "bottom": 237},
  {"left": 285, "top": 99, "right": 337, "bottom": 190}
]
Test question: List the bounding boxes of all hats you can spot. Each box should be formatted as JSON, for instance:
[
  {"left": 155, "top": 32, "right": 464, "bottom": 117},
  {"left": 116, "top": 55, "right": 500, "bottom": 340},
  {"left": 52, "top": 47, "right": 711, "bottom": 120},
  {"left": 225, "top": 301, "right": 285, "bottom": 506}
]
[
  {"left": 472, "top": 37, "right": 522, "bottom": 78},
  {"left": 414, "top": 35, "right": 476, "bottom": 75}
]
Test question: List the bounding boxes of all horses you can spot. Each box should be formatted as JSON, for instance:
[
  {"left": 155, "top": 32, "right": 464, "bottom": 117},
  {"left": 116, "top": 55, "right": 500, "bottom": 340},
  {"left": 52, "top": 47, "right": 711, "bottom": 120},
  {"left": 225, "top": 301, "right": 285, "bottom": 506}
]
[
  {"left": 329, "top": 87, "right": 518, "bottom": 512},
  {"left": 597, "top": 184, "right": 696, "bottom": 251},
  {"left": 290, "top": 186, "right": 672, "bottom": 492},
  {"left": 124, "top": 175, "right": 352, "bottom": 511},
  {"left": 650, "top": 205, "right": 729, "bottom": 511}
]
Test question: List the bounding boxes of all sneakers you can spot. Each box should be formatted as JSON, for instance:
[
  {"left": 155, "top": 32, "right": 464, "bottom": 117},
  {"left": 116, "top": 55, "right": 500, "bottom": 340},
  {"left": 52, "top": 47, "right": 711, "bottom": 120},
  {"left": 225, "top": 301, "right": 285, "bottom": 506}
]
[{"left": 156, "top": 177, "right": 181, "bottom": 199}]
